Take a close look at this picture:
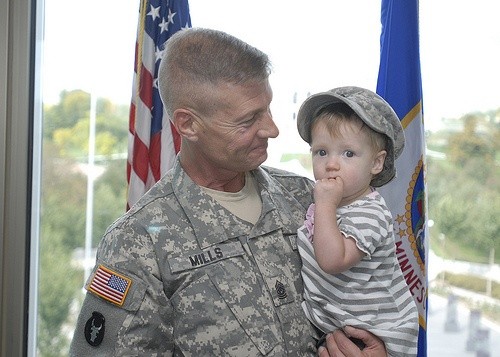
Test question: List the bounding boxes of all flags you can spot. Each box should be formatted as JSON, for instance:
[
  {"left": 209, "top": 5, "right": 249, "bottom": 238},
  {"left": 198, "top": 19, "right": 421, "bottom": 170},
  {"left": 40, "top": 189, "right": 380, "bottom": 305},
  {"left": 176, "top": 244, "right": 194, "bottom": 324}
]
[
  {"left": 375, "top": 0, "right": 429, "bottom": 357},
  {"left": 125, "top": 0, "right": 193, "bottom": 212}
]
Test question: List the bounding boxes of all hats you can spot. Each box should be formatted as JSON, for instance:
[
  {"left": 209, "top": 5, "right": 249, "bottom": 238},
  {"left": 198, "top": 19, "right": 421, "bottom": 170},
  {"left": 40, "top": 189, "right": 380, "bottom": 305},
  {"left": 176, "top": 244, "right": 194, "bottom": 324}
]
[{"left": 297, "top": 86, "right": 406, "bottom": 189}]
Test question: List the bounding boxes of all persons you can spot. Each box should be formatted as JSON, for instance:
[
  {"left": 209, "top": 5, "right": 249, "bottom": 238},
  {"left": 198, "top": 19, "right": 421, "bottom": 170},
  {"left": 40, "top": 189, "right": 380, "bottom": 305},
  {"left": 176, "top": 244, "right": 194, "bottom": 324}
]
[
  {"left": 65, "top": 27, "right": 388, "bottom": 357},
  {"left": 297, "top": 87, "right": 419, "bottom": 357}
]
